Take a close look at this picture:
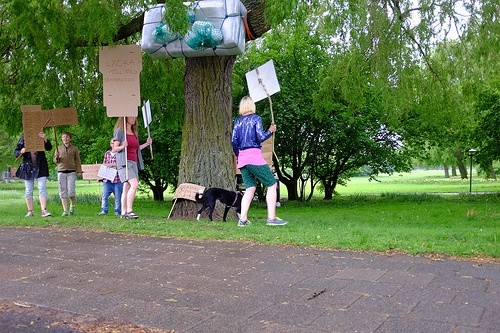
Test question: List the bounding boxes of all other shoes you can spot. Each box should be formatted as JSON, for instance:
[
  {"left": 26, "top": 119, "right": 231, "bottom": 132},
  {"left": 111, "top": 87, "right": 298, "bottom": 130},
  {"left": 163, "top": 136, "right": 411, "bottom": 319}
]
[
  {"left": 69, "top": 209, "right": 74, "bottom": 215},
  {"left": 99, "top": 210, "right": 107, "bottom": 216},
  {"left": 114, "top": 212, "right": 120, "bottom": 216},
  {"left": 62, "top": 211, "right": 68, "bottom": 216}
]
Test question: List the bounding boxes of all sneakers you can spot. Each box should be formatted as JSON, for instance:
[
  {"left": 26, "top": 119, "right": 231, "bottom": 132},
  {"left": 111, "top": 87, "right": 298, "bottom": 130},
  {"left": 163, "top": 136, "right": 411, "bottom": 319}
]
[
  {"left": 41, "top": 208, "right": 51, "bottom": 217},
  {"left": 237, "top": 219, "right": 254, "bottom": 226},
  {"left": 266, "top": 217, "right": 288, "bottom": 226},
  {"left": 25, "top": 210, "right": 34, "bottom": 217}
]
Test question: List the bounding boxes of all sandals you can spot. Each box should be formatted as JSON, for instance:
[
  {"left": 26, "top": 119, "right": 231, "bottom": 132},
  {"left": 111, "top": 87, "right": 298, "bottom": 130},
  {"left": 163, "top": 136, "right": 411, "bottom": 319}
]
[{"left": 120, "top": 210, "right": 139, "bottom": 219}]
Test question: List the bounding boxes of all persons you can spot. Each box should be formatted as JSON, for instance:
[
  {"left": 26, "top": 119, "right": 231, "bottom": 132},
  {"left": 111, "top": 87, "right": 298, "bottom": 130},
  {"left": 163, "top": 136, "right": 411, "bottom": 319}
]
[
  {"left": 232, "top": 97, "right": 289, "bottom": 227},
  {"left": 53, "top": 132, "right": 82, "bottom": 216},
  {"left": 14, "top": 132, "right": 53, "bottom": 217},
  {"left": 99, "top": 139, "right": 122, "bottom": 216},
  {"left": 113, "top": 116, "right": 152, "bottom": 219}
]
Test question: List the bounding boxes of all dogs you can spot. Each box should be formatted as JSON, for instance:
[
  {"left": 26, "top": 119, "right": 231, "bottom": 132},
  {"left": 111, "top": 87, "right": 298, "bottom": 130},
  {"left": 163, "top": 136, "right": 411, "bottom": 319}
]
[{"left": 195, "top": 188, "right": 245, "bottom": 223}]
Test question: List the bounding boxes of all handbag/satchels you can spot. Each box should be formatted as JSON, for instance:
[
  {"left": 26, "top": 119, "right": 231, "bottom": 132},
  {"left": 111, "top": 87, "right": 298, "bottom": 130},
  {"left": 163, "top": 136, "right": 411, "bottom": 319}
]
[{"left": 18, "top": 162, "right": 33, "bottom": 180}]
